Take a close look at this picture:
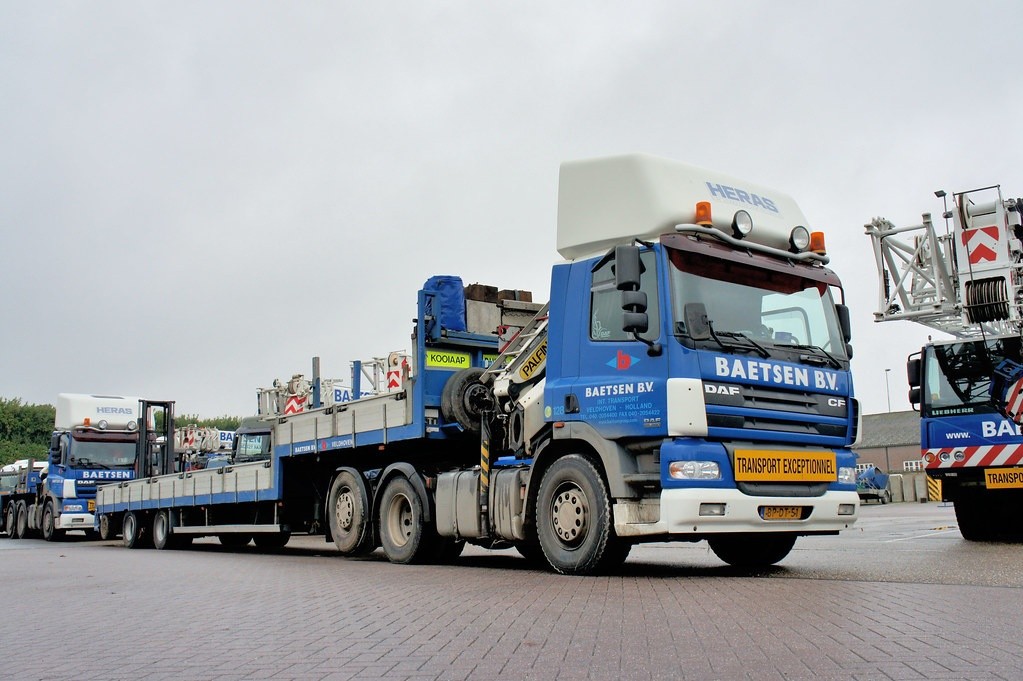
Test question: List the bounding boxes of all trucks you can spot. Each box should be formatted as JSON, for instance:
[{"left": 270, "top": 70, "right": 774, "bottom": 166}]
[{"left": 863, "top": 186, "right": 1023, "bottom": 542}]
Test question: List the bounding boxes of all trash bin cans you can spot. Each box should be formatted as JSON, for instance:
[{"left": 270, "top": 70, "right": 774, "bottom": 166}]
[
  {"left": 915, "top": 473, "right": 928, "bottom": 502},
  {"left": 903, "top": 473, "right": 916, "bottom": 502},
  {"left": 890, "top": 474, "right": 904, "bottom": 502}
]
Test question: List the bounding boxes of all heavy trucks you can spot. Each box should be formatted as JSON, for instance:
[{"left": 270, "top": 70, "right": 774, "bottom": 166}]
[
  {"left": 0, "top": 391, "right": 156, "bottom": 542},
  {"left": 88, "top": 152, "right": 868, "bottom": 575}
]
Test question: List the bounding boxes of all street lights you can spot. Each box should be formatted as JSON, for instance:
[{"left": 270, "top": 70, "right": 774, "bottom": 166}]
[{"left": 883, "top": 368, "right": 891, "bottom": 413}]
